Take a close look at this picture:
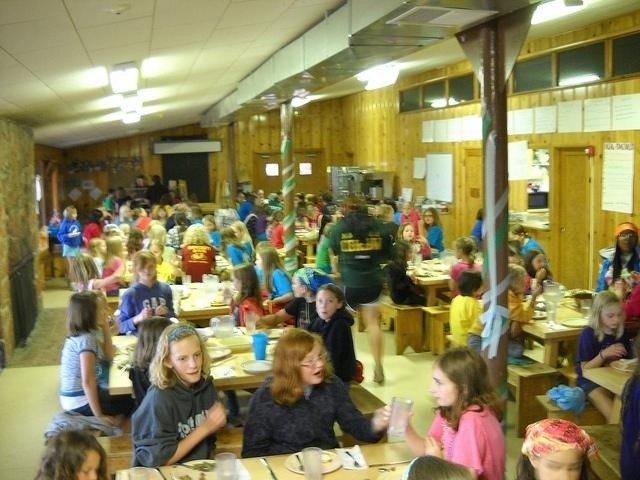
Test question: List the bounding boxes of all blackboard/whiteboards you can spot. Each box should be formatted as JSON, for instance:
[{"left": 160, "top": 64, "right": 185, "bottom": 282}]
[{"left": 425, "top": 153, "right": 454, "bottom": 204}]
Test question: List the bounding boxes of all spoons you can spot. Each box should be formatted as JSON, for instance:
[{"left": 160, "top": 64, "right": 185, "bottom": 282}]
[{"left": 295, "top": 454, "right": 304, "bottom": 471}]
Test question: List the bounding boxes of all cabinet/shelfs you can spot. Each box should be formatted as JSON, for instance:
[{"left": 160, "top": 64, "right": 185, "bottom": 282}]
[
  {"left": 330, "top": 165, "right": 363, "bottom": 202},
  {"left": 63, "top": 172, "right": 108, "bottom": 216}
]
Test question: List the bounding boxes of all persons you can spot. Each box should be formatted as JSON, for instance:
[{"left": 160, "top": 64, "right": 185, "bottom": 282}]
[
  {"left": 58, "top": 177, "right": 176, "bottom": 332},
  {"left": 576, "top": 290, "right": 636, "bottom": 425},
  {"left": 165, "top": 187, "right": 333, "bottom": 332},
  {"left": 58, "top": 289, "right": 135, "bottom": 427},
  {"left": 399, "top": 454, "right": 474, "bottom": 480},
  {"left": 128, "top": 322, "right": 229, "bottom": 468},
  {"left": 612, "top": 269, "right": 640, "bottom": 321},
  {"left": 448, "top": 209, "right": 552, "bottom": 358},
  {"left": 33, "top": 428, "right": 110, "bottom": 480},
  {"left": 619, "top": 363, "right": 640, "bottom": 480},
  {"left": 382, "top": 348, "right": 507, "bottom": 480},
  {"left": 594, "top": 221, "right": 640, "bottom": 292},
  {"left": 295, "top": 189, "right": 443, "bottom": 382},
  {"left": 511, "top": 418, "right": 602, "bottom": 480},
  {"left": 240, "top": 327, "right": 393, "bottom": 460}
]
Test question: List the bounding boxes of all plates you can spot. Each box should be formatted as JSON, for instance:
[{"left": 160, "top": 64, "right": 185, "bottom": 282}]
[
  {"left": 407, "top": 265, "right": 415, "bottom": 270},
  {"left": 242, "top": 360, "right": 273, "bottom": 374},
  {"left": 198, "top": 328, "right": 214, "bottom": 337},
  {"left": 416, "top": 272, "right": 428, "bottom": 277},
  {"left": 532, "top": 310, "right": 547, "bottom": 318},
  {"left": 208, "top": 349, "right": 231, "bottom": 359},
  {"left": 267, "top": 329, "right": 282, "bottom": 339},
  {"left": 564, "top": 319, "right": 590, "bottom": 327},
  {"left": 171, "top": 459, "right": 219, "bottom": 480},
  {"left": 286, "top": 450, "right": 342, "bottom": 475},
  {"left": 611, "top": 360, "right": 636, "bottom": 372},
  {"left": 223, "top": 336, "right": 250, "bottom": 352}
]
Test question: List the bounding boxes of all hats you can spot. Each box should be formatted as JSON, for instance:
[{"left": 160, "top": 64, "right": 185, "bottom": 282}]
[{"left": 613, "top": 221, "right": 639, "bottom": 237}]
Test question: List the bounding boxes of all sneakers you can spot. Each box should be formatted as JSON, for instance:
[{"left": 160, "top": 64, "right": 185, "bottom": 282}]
[{"left": 372, "top": 365, "right": 385, "bottom": 384}]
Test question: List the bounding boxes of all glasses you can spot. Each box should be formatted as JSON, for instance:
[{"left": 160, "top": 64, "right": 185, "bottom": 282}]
[{"left": 619, "top": 232, "right": 636, "bottom": 239}]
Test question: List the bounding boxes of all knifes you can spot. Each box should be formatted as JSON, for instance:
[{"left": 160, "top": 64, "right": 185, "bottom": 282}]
[
  {"left": 260, "top": 458, "right": 277, "bottom": 480},
  {"left": 369, "top": 461, "right": 410, "bottom": 468}
]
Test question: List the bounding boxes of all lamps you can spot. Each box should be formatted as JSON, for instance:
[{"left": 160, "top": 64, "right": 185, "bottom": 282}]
[
  {"left": 108, "top": 59, "right": 146, "bottom": 126},
  {"left": 531, "top": 0, "right": 584, "bottom": 22}
]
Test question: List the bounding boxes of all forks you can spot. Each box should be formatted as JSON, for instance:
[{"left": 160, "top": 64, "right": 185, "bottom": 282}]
[{"left": 345, "top": 452, "right": 362, "bottom": 468}]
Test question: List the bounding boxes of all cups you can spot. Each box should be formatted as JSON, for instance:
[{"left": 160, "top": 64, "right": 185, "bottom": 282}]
[
  {"left": 214, "top": 453, "right": 237, "bottom": 480},
  {"left": 386, "top": 396, "right": 413, "bottom": 437},
  {"left": 181, "top": 275, "right": 192, "bottom": 292},
  {"left": 246, "top": 311, "right": 256, "bottom": 332},
  {"left": 129, "top": 467, "right": 150, "bottom": 480},
  {"left": 542, "top": 280, "right": 551, "bottom": 292},
  {"left": 252, "top": 332, "right": 267, "bottom": 360},
  {"left": 302, "top": 447, "right": 322, "bottom": 480}
]
